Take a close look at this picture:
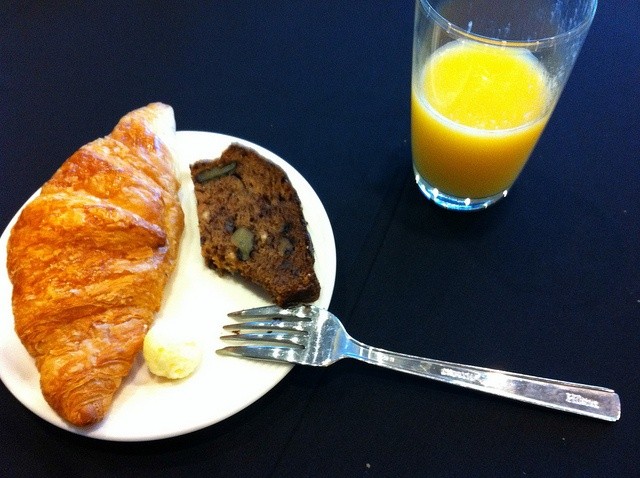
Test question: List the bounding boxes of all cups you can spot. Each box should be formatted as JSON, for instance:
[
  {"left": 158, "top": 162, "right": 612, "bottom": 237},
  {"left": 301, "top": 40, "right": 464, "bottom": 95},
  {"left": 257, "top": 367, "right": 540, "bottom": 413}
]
[{"left": 412, "top": 0, "right": 598, "bottom": 210}]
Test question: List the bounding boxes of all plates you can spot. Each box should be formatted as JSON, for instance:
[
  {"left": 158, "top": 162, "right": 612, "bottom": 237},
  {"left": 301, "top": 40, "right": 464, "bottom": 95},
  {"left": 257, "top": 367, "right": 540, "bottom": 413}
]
[{"left": 3, "top": 130, "right": 338, "bottom": 441}]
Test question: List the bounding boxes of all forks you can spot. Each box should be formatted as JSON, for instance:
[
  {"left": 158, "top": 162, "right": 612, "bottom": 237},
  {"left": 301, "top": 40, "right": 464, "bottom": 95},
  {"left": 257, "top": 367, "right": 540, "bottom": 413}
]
[{"left": 216, "top": 301, "right": 623, "bottom": 424}]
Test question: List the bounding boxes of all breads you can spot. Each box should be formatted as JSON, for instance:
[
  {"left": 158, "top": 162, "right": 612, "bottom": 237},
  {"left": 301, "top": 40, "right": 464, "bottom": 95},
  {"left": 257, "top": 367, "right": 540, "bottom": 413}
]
[
  {"left": 190, "top": 143, "right": 320, "bottom": 307},
  {"left": 6, "top": 101, "right": 183, "bottom": 426}
]
